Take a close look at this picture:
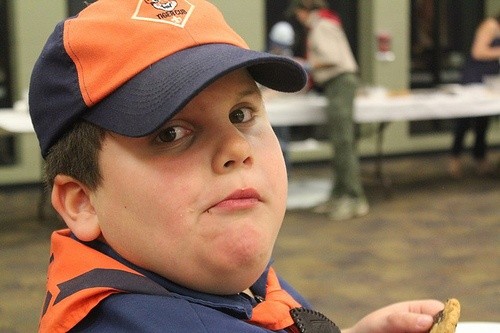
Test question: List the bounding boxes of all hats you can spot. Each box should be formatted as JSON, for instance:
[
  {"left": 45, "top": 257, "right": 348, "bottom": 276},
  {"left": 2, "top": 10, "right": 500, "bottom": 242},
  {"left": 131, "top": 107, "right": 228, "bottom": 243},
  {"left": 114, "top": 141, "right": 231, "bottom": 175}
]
[{"left": 27, "top": 0, "right": 309, "bottom": 158}]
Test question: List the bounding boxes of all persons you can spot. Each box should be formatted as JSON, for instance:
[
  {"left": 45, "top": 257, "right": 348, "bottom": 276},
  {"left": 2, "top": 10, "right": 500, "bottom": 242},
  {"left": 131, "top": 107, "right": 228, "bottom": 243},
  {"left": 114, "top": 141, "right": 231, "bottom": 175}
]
[
  {"left": 293, "top": 0, "right": 370, "bottom": 222},
  {"left": 447, "top": 11, "right": 500, "bottom": 176},
  {"left": 29, "top": 0, "right": 445, "bottom": 333}
]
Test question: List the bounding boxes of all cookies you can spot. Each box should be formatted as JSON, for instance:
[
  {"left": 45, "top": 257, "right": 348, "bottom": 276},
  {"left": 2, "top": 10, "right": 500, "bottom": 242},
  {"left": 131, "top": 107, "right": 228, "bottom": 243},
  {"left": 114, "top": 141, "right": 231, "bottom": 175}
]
[{"left": 428, "top": 298, "right": 460, "bottom": 333}]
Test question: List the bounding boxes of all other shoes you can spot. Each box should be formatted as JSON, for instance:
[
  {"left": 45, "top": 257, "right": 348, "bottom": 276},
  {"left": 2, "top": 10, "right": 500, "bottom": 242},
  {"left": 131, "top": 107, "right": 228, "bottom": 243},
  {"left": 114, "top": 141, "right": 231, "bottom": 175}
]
[{"left": 330, "top": 195, "right": 372, "bottom": 221}]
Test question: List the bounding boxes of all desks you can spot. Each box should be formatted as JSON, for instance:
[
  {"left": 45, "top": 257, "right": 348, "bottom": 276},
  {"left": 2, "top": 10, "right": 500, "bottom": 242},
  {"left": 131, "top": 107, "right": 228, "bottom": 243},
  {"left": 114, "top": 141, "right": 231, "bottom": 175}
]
[{"left": 0, "top": 89, "right": 500, "bottom": 220}]
